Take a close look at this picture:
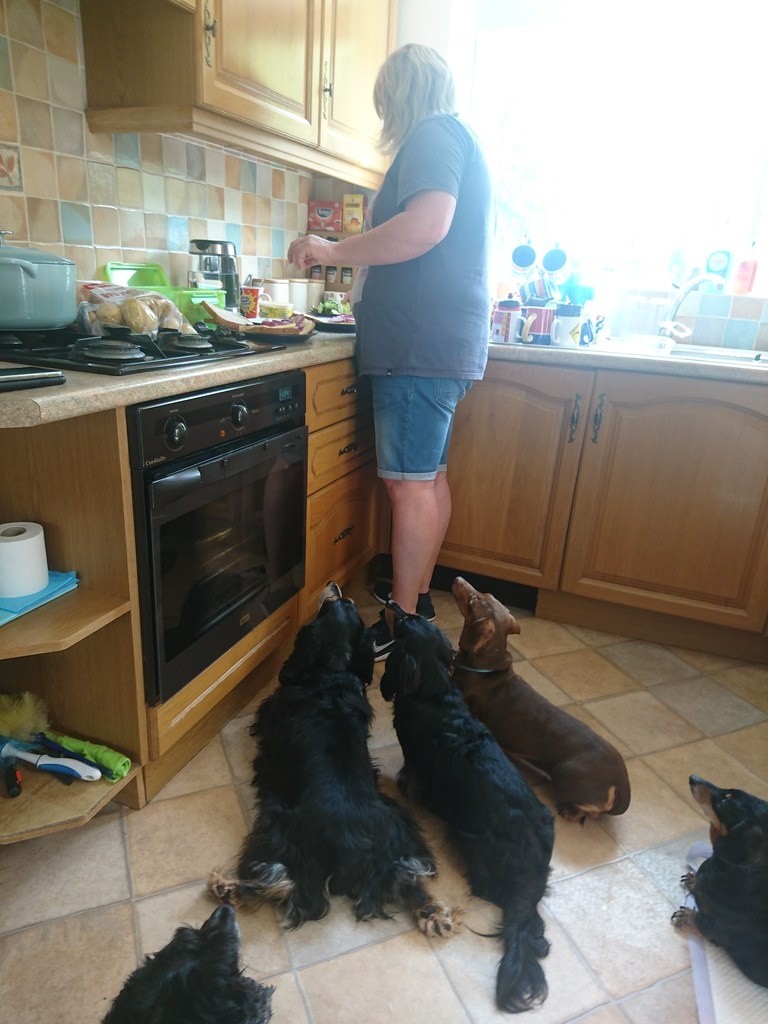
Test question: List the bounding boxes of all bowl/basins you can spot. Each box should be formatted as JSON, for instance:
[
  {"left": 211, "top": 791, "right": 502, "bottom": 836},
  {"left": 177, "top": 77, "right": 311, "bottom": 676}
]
[{"left": 259, "top": 300, "right": 293, "bottom": 320}]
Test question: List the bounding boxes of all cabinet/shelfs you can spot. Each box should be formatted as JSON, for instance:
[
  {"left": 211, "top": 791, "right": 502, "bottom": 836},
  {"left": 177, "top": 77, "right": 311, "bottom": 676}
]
[
  {"left": 80, "top": 0, "right": 398, "bottom": 192},
  {"left": 0, "top": 406, "right": 148, "bottom": 846},
  {"left": 303, "top": 358, "right": 768, "bottom": 665}
]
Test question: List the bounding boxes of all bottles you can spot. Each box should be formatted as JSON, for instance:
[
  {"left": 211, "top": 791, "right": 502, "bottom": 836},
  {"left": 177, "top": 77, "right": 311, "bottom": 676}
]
[
  {"left": 341, "top": 267, "right": 352, "bottom": 284},
  {"left": 327, "top": 236, "right": 337, "bottom": 242},
  {"left": 253, "top": 278, "right": 325, "bottom": 312},
  {"left": 736, "top": 240, "right": 758, "bottom": 292},
  {"left": 311, "top": 265, "right": 321, "bottom": 279},
  {"left": 325, "top": 266, "right": 336, "bottom": 283}
]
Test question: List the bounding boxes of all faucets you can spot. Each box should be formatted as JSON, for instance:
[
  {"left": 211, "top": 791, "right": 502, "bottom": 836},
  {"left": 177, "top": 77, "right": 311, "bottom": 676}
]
[{"left": 657, "top": 273, "right": 725, "bottom": 352}]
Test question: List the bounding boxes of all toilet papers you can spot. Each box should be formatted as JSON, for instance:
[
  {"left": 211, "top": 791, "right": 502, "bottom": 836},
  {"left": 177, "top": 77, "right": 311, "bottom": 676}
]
[{"left": 0, "top": 522, "right": 51, "bottom": 600}]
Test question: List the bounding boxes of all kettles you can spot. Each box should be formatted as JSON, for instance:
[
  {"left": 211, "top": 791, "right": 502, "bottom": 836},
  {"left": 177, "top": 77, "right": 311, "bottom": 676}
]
[{"left": 188, "top": 239, "right": 240, "bottom": 314}]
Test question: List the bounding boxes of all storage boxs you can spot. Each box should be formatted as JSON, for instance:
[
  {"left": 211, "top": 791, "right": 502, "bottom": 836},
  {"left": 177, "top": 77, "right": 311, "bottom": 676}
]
[
  {"left": 307, "top": 231, "right": 358, "bottom": 293},
  {"left": 343, "top": 195, "right": 368, "bottom": 233},
  {"left": 104, "top": 261, "right": 228, "bottom": 332},
  {"left": 309, "top": 200, "right": 343, "bottom": 232}
]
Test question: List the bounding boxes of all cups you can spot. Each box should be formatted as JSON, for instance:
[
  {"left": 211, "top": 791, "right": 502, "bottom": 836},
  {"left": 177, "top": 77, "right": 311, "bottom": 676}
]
[
  {"left": 323, "top": 291, "right": 347, "bottom": 306},
  {"left": 550, "top": 316, "right": 580, "bottom": 349},
  {"left": 540, "top": 242, "right": 569, "bottom": 284},
  {"left": 489, "top": 306, "right": 555, "bottom": 345},
  {"left": 240, "top": 286, "right": 272, "bottom": 318},
  {"left": 520, "top": 266, "right": 553, "bottom": 304},
  {"left": 511, "top": 240, "right": 537, "bottom": 277}
]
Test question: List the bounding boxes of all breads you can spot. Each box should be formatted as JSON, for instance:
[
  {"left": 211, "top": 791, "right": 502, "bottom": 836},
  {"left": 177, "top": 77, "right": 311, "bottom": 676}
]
[{"left": 200, "top": 300, "right": 316, "bottom": 336}]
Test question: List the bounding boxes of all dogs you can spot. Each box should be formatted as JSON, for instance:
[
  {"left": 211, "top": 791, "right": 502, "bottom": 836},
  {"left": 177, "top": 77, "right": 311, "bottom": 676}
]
[
  {"left": 203, "top": 580, "right": 467, "bottom": 941},
  {"left": 380, "top": 577, "right": 630, "bottom": 1015},
  {"left": 100, "top": 902, "right": 277, "bottom": 1024},
  {"left": 671, "top": 775, "right": 768, "bottom": 988}
]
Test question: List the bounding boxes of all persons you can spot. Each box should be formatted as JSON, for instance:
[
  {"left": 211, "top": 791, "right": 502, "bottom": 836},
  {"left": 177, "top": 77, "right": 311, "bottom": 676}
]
[{"left": 287, "top": 43, "right": 492, "bottom": 661}]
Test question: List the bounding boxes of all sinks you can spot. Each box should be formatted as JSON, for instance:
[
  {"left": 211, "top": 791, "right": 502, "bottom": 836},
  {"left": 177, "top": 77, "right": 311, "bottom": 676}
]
[{"left": 670, "top": 348, "right": 768, "bottom": 367}]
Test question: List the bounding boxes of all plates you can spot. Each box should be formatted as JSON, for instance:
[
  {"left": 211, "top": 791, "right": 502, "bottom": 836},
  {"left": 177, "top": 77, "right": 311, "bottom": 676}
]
[
  {"left": 243, "top": 329, "right": 318, "bottom": 342},
  {"left": 314, "top": 323, "right": 355, "bottom": 333}
]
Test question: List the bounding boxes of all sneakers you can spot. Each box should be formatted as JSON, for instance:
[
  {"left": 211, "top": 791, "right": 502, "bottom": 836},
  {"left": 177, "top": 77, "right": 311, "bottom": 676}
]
[
  {"left": 373, "top": 581, "right": 436, "bottom": 622},
  {"left": 367, "top": 601, "right": 408, "bottom": 662}
]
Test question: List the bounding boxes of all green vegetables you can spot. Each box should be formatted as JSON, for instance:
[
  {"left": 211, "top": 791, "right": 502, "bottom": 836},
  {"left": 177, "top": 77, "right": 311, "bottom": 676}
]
[{"left": 308, "top": 299, "right": 356, "bottom": 316}]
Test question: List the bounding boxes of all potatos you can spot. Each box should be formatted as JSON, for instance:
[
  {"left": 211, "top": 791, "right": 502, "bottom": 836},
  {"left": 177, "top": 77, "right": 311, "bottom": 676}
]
[{"left": 75, "top": 281, "right": 197, "bottom": 335}]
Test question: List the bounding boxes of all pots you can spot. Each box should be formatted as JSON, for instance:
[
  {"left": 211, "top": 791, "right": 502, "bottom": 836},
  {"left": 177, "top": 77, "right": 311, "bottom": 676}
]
[{"left": 0, "top": 231, "right": 76, "bottom": 330}]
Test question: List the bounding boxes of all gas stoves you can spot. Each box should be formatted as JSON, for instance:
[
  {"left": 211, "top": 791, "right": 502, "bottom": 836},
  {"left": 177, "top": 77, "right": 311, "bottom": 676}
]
[{"left": 0, "top": 322, "right": 287, "bottom": 376}]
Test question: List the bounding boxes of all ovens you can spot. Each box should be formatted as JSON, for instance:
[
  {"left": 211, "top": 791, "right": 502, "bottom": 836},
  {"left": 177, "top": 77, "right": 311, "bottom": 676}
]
[{"left": 135, "top": 370, "right": 310, "bottom": 707}]
